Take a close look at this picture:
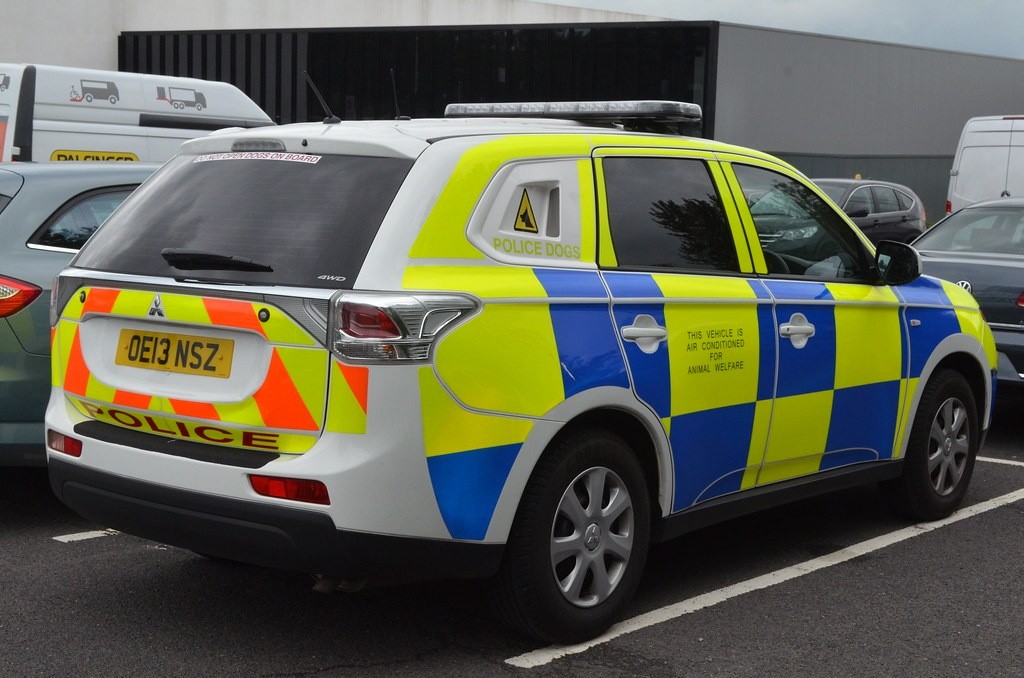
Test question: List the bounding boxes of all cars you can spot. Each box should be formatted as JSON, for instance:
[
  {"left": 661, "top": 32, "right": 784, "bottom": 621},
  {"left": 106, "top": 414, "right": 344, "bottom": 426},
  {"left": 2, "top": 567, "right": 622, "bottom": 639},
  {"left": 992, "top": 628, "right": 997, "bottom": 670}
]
[
  {"left": 909, "top": 199, "right": 1022, "bottom": 397},
  {"left": 0, "top": 162, "right": 169, "bottom": 504},
  {"left": 43, "top": 101, "right": 998, "bottom": 644},
  {"left": 748, "top": 178, "right": 927, "bottom": 262}
]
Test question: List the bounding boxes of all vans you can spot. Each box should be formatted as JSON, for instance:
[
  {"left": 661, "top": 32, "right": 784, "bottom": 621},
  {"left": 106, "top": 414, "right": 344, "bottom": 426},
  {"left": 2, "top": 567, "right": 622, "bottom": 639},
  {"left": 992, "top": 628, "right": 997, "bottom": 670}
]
[
  {"left": 946, "top": 113, "right": 1024, "bottom": 216},
  {"left": 0, "top": 62, "right": 279, "bottom": 165}
]
